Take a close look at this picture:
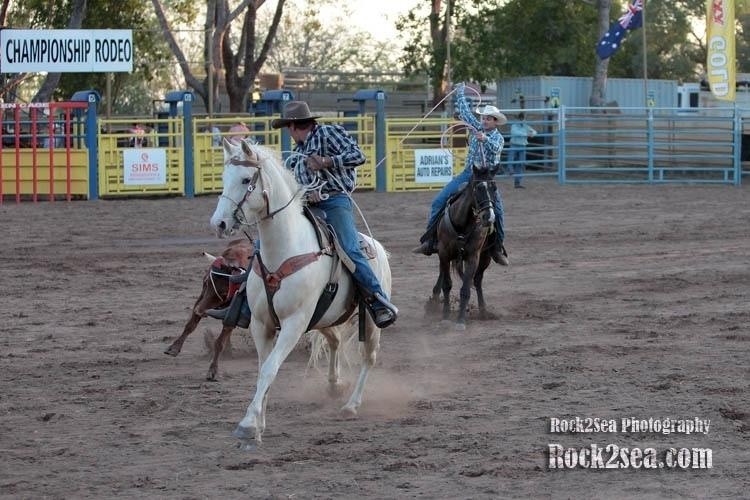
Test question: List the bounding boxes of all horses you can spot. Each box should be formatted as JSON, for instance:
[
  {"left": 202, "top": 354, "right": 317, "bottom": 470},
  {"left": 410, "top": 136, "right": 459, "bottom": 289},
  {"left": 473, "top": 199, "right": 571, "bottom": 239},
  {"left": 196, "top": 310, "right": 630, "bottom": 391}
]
[
  {"left": 209, "top": 137, "right": 391, "bottom": 449},
  {"left": 433, "top": 163, "right": 499, "bottom": 332}
]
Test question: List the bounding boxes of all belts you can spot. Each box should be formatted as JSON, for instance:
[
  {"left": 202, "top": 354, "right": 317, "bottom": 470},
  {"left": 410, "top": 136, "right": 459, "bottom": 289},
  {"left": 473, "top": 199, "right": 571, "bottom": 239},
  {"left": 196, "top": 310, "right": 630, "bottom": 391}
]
[{"left": 320, "top": 188, "right": 347, "bottom": 200}]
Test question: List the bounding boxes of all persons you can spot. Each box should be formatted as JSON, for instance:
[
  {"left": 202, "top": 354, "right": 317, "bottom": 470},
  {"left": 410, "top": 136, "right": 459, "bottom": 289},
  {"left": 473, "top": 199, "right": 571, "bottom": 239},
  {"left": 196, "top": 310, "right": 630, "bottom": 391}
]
[
  {"left": 227, "top": 116, "right": 250, "bottom": 142},
  {"left": 43, "top": 124, "right": 62, "bottom": 148},
  {"left": 127, "top": 123, "right": 145, "bottom": 148},
  {"left": 411, "top": 82, "right": 510, "bottom": 267},
  {"left": 205, "top": 126, "right": 222, "bottom": 149},
  {"left": 203, "top": 100, "right": 395, "bottom": 329},
  {"left": 505, "top": 111, "right": 539, "bottom": 191},
  {"left": 143, "top": 123, "right": 160, "bottom": 147}
]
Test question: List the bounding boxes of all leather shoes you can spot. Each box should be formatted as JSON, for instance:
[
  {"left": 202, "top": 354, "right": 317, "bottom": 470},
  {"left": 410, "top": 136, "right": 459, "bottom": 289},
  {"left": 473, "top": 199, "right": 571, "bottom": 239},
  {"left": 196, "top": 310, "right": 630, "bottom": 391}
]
[
  {"left": 365, "top": 296, "right": 395, "bottom": 329},
  {"left": 487, "top": 247, "right": 511, "bottom": 266},
  {"left": 203, "top": 303, "right": 250, "bottom": 329},
  {"left": 410, "top": 240, "right": 433, "bottom": 256}
]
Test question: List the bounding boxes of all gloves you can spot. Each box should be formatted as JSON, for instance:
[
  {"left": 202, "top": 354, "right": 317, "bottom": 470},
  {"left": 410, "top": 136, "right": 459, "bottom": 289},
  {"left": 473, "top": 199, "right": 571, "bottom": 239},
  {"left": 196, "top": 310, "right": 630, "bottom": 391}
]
[{"left": 306, "top": 153, "right": 335, "bottom": 171}]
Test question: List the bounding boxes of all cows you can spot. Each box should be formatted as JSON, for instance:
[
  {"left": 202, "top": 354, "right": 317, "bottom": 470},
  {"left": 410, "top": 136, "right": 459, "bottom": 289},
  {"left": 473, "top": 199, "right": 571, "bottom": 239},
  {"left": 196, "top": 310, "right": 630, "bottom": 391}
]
[{"left": 163, "top": 239, "right": 256, "bottom": 381}]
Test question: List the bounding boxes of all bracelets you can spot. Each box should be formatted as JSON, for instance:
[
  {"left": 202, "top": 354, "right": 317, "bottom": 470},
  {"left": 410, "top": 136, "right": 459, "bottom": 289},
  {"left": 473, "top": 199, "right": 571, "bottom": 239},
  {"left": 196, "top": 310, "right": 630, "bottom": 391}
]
[{"left": 321, "top": 156, "right": 326, "bottom": 167}]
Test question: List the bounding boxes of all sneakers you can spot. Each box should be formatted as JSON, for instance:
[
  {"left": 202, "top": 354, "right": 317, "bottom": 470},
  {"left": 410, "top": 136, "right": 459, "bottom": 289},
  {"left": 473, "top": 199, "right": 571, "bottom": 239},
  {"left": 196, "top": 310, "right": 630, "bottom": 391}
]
[{"left": 514, "top": 182, "right": 527, "bottom": 188}]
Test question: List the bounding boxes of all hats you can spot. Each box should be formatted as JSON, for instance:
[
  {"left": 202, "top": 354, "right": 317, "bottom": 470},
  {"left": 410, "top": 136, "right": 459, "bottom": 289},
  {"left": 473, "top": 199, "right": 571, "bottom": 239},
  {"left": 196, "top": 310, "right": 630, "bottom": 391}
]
[
  {"left": 271, "top": 98, "right": 322, "bottom": 129},
  {"left": 476, "top": 104, "right": 508, "bottom": 126}
]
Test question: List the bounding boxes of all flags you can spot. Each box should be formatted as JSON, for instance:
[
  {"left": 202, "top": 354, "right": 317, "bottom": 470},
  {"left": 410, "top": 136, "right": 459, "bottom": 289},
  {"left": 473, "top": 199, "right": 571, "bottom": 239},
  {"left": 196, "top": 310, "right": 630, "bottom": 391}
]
[{"left": 596, "top": 0, "right": 643, "bottom": 59}]
